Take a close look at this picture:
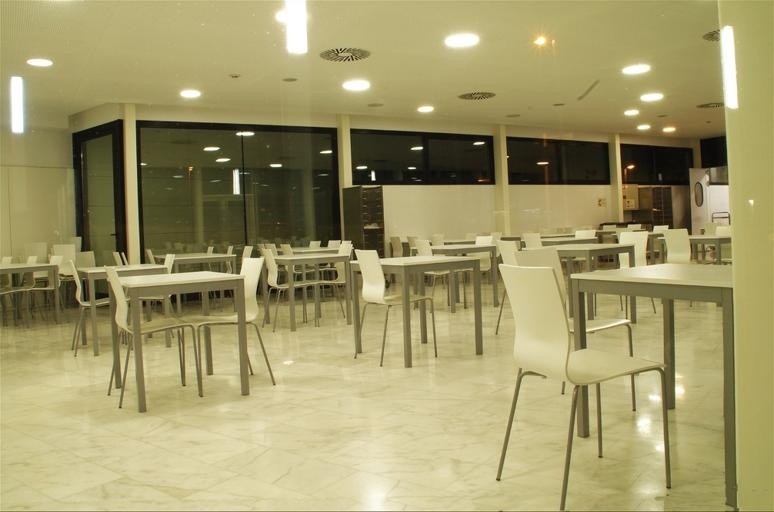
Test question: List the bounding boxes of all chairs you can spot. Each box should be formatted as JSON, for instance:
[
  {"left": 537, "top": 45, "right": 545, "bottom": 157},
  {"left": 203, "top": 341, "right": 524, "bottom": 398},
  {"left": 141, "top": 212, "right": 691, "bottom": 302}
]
[{"left": 492, "top": 255, "right": 674, "bottom": 511}]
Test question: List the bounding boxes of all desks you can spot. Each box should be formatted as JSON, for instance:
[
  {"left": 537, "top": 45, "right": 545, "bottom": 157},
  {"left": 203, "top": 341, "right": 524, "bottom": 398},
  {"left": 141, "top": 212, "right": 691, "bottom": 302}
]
[{"left": 564, "top": 264, "right": 737, "bottom": 511}]
